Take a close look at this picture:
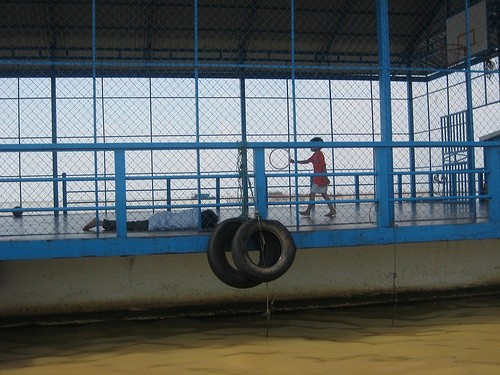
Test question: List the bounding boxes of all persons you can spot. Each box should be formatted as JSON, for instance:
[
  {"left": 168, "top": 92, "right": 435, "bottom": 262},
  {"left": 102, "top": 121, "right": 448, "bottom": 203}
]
[
  {"left": 290, "top": 137, "right": 336, "bottom": 216},
  {"left": 83, "top": 209, "right": 218, "bottom": 231}
]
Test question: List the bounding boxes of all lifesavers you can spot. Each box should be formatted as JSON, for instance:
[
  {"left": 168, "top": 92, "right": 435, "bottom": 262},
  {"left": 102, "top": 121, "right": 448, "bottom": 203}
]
[
  {"left": 231, "top": 219, "right": 297, "bottom": 283},
  {"left": 207, "top": 217, "right": 263, "bottom": 289}
]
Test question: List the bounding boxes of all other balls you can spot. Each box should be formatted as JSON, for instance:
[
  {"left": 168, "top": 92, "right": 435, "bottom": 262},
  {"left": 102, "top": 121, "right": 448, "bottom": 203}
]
[{"left": 13, "top": 206, "right": 23, "bottom": 217}]
